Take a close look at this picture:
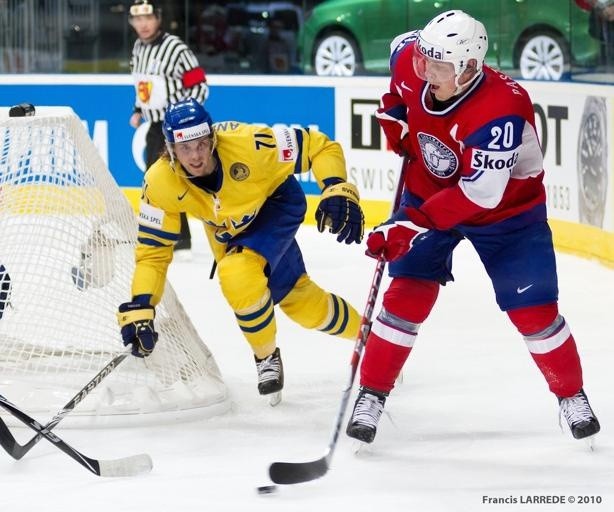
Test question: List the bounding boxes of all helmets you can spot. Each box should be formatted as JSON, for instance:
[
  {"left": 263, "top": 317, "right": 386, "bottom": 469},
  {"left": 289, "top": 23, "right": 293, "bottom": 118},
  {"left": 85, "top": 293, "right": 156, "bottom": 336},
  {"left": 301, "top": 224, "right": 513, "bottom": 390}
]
[
  {"left": 160, "top": 97, "right": 216, "bottom": 148},
  {"left": 411, "top": 6, "right": 491, "bottom": 99},
  {"left": 127, "top": 1, "right": 161, "bottom": 16}
]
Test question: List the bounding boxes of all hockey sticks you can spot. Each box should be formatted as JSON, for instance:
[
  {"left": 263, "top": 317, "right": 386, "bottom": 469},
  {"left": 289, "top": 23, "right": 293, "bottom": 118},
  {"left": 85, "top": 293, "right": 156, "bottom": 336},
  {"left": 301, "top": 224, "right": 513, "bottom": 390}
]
[
  {"left": 1, "top": 397, "right": 153, "bottom": 476},
  {"left": 1, "top": 345, "right": 133, "bottom": 459},
  {"left": 269, "top": 158, "right": 406, "bottom": 484}
]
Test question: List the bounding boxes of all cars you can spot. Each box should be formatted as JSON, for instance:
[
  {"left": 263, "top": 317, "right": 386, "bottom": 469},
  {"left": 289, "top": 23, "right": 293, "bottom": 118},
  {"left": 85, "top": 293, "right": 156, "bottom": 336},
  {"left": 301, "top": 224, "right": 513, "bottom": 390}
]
[
  {"left": 296, "top": 1, "right": 608, "bottom": 82},
  {"left": 224, "top": 4, "right": 306, "bottom": 69}
]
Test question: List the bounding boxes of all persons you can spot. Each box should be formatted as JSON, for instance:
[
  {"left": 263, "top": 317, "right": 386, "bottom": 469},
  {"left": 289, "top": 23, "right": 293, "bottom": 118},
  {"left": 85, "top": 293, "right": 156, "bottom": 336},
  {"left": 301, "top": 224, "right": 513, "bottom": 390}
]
[
  {"left": 126, "top": 1, "right": 210, "bottom": 251},
  {"left": 344, "top": 8, "right": 600, "bottom": 443},
  {"left": 116, "top": 99, "right": 372, "bottom": 397}
]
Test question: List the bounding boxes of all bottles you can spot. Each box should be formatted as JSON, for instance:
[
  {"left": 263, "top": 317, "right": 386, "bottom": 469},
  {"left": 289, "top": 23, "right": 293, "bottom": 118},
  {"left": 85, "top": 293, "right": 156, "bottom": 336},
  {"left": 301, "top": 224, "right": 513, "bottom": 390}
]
[{"left": 10, "top": 102, "right": 38, "bottom": 118}]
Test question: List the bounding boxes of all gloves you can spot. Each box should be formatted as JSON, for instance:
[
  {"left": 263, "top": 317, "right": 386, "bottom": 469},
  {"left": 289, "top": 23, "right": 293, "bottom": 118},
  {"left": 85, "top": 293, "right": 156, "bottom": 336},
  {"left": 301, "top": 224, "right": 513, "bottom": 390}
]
[
  {"left": 365, "top": 206, "right": 437, "bottom": 263},
  {"left": 315, "top": 182, "right": 365, "bottom": 246},
  {"left": 115, "top": 301, "right": 159, "bottom": 359},
  {"left": 374, "top": 93, "right": 418, "bottom": 162}
]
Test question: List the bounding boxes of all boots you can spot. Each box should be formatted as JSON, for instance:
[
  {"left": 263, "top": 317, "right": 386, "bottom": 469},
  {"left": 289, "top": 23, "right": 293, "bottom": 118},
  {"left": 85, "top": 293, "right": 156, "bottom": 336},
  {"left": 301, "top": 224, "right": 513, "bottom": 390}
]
[
  {"left": 342, "top": 384, "right": 390, "bottom": 445},
  {"left": 252, "top": 346, "right": 286, "bottom": 397},
  {"left": 555, "top": 387, "right": 602, "bottom": 442}
]
